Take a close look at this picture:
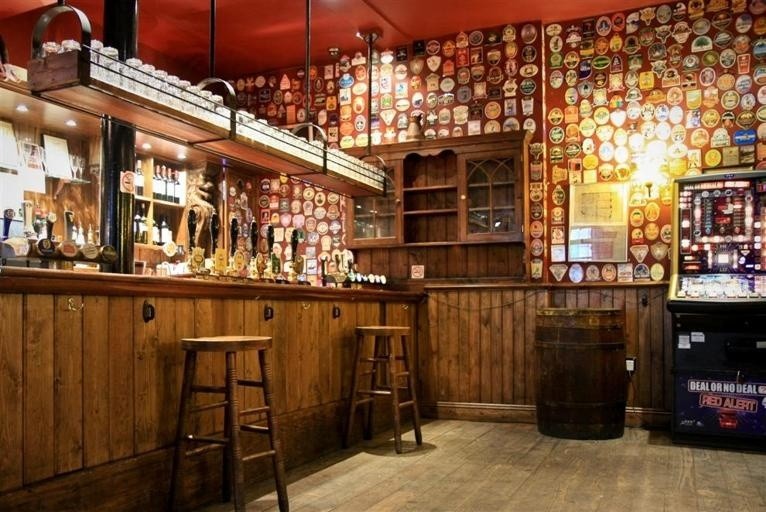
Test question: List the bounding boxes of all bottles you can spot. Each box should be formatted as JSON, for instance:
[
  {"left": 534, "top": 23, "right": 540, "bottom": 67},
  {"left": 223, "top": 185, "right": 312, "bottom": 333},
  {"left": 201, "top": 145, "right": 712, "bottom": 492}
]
[
  {"left": 135, "top": 157, "right": 181, "bottom": 203},
  {"left": 131, "top": 204, "right": 174, "bottom": 244}
]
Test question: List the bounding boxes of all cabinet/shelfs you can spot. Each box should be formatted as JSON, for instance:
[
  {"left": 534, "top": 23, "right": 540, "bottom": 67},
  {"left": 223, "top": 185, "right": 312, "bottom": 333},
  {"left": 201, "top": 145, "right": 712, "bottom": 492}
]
[
  {"left": 0, "top": 0, "right": 386, "bottom": 272},
  {"left": 335, "top": 128, "right": 534, "bottom": 279}
]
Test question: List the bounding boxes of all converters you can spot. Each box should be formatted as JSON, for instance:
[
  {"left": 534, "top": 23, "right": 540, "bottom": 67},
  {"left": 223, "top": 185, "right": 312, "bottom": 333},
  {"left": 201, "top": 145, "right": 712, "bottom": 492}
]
[{"left": 626, "top": 360, "right": 635, "bottom": 373}]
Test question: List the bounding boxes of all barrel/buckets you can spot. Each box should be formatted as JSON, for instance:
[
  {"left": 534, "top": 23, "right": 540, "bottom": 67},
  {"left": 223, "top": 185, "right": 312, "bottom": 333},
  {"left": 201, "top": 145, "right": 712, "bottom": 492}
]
[{"left": 532, "top": 307, "right": 628, "bottom": 439}]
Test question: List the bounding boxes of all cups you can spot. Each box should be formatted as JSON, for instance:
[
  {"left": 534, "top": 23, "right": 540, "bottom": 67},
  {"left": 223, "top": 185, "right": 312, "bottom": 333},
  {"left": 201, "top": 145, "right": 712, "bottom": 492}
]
[{"left": 41, "top": 40, "right": 385, "bottom": 190}]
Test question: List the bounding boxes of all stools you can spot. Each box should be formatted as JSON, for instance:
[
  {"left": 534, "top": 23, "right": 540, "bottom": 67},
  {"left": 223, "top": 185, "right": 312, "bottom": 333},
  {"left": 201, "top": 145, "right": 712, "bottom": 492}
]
[
  {"left": 168, "top": 335, "right": 290, "bottom": 510},
  {"left": 346, "top": 326, "right": 422, "bottom": 453}
]
